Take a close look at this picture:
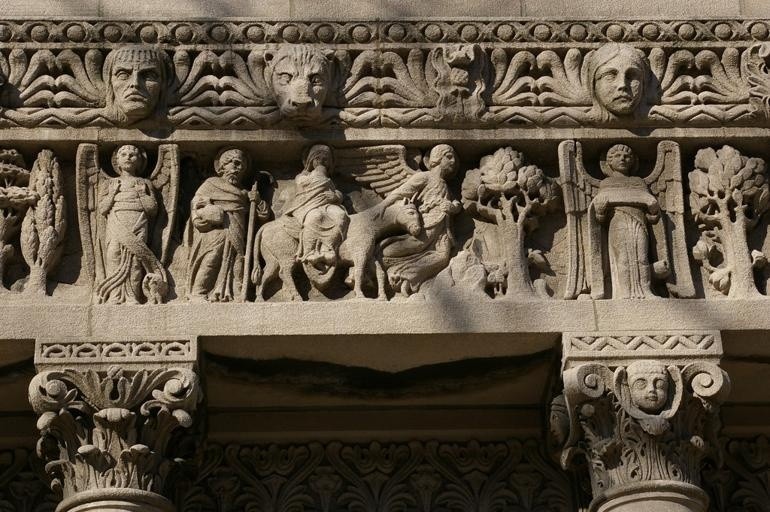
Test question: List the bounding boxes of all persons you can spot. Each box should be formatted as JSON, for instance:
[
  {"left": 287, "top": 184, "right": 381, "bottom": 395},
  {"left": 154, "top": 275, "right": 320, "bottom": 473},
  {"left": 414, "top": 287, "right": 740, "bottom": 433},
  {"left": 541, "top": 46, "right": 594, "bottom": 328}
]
[
  {"left": 625, "top": 360, "right": 669, "bottom": 411},
  {"left": 593, "top": 144, "right": 661, "bottom": 301},
  {"left": 586, "top": 42, "right": 648, "bottom": 122},
  {"left": 270, "top": 144, "right": 350, "bottom": 264},
  {"left": 104, "top": 45, "right": 168, "bottom": 122},
  {"left": 344, "top": 144, "right": 463, "bottom": 297},
  {"left": 182, "top": 145, "right": 272, "bottom": 303},
  {"left": 550, "top": 394, "right": 570, "bottom": 445},
  {"left": 97, "top": 145, "right": 159, "bottom": 305}
]
